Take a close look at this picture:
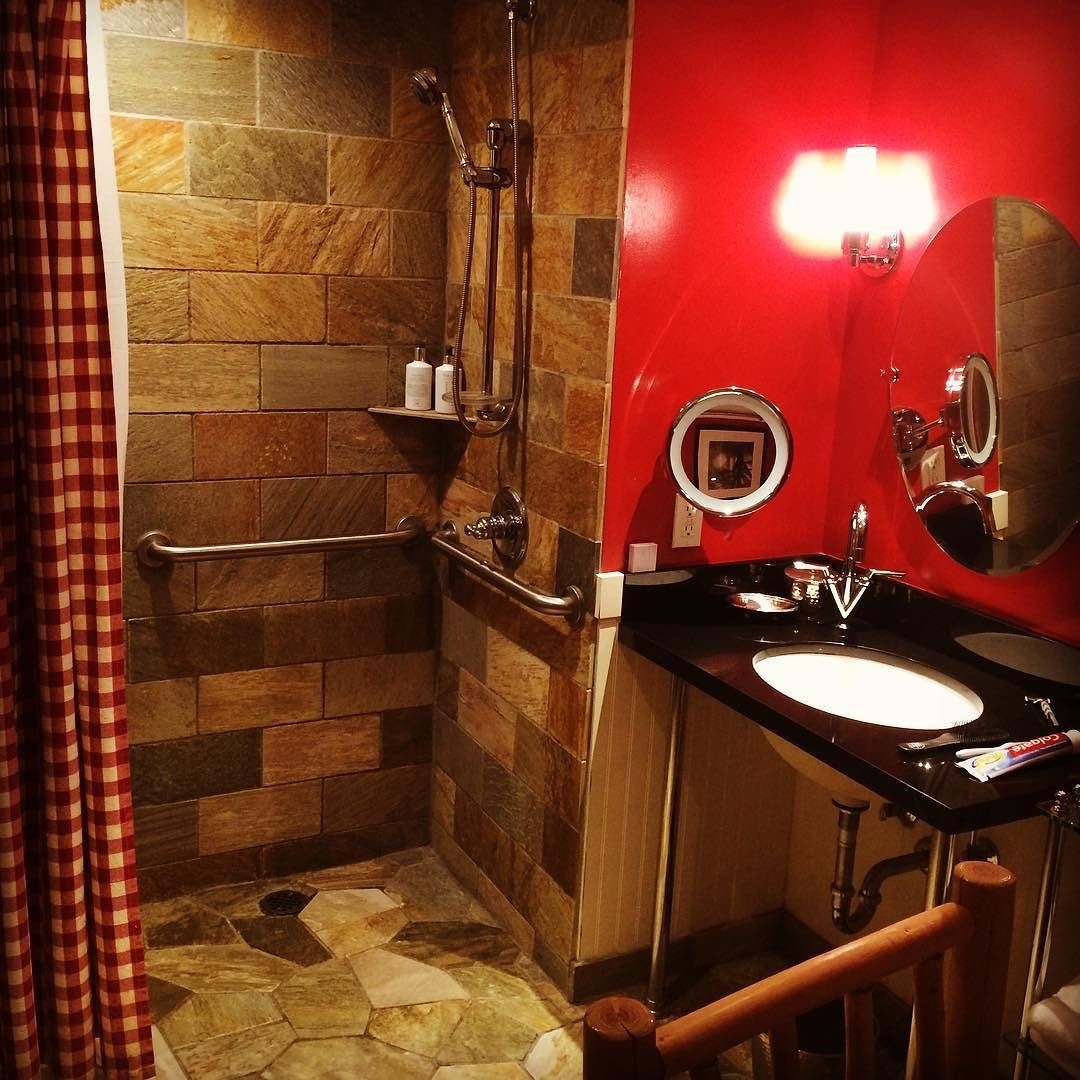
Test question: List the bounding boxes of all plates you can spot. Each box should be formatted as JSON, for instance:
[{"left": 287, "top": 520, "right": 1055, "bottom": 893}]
[{"left": 729, "top": 590, "right": 800, "bottom": 623}]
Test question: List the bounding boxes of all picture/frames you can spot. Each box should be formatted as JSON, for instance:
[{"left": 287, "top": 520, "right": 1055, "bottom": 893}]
[{"left": 695, "top": 424, "right": 769, "bottom": 501}]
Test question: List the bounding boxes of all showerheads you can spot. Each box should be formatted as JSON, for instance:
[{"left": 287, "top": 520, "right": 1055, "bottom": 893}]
[{"left": 406, "top": 63, "right": 479, "bottom": 184}]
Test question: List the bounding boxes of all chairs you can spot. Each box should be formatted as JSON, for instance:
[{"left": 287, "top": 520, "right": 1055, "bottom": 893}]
[{"left": 580, "top": 860, "right": 1025, "bottom": 1080}]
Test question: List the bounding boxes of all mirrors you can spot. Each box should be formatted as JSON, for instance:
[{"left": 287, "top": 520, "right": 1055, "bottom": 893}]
[
  {"left": 891, "top": 350, "right": 1001, "bottom": 472},
  {"left": 888, "top": 194, "right": 1079, "bottom": 581},
  {"left": 666, "top": 385, "right": 794, "bottom": 521}
]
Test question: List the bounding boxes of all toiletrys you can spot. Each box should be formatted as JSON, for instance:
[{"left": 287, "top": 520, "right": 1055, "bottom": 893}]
[
  {"left": 955, "top": 729, "right": 1080, "bottom": 785},
  {"left": 403, "top": 340, "right": 464, "bottom": 418}
]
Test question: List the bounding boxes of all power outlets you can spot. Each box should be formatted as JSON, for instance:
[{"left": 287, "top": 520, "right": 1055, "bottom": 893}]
[
  {"left": 670, "top": 491, "right": 704, "bottom": 550},
  {"left": 921, "top": 444, "right": 947, "bottom": 496}
]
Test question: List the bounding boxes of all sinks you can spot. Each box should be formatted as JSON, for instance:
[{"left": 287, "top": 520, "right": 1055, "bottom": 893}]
[{"left": 752, "top": 641, "right": 985, "bottom": 803}]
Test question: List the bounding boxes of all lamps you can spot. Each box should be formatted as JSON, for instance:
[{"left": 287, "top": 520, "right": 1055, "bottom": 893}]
[{"left": 772, "top": 143, "right": 935, "bottom": 276}]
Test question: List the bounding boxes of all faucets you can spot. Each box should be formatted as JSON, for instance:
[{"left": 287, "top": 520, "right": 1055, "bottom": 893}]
[{"left": 840, "top": 499, "right": 869, "bottom": 636}]
